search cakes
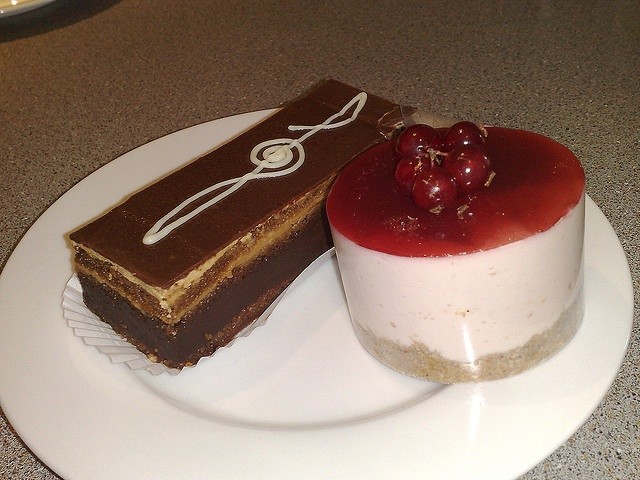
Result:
[69,78,418,371]
[325,120,587,386]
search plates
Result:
[1,109,635,479]
